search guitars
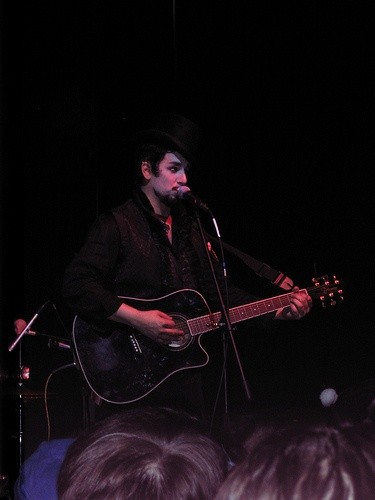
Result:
[70,274,347,406]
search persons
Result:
[56,398,375,500]
[62,132,314,414]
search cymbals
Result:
[0,386,64,400]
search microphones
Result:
[177,186,209,211]
[9,305,43,351]
